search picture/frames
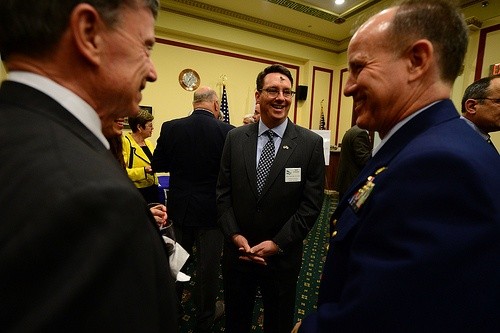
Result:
[115,106,152,129]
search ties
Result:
[256,130,276,196]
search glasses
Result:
[473,97,500,103]
[258,88,297,98]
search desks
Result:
[325,147,342,189]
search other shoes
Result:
[180,287,192,306]
[196,300,224,326]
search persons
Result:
[333,124,373,201]
[156,84,237,333]
[118,106,161,205]
[100,114,167,231]
[215,63,326,333]
[0,0,186,332]
[290,0,500,332]
[253,104,261,123]
[243,114,254,124]
[460,75,499,131]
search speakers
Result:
[297,86,308,100]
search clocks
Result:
[179,68,201,91]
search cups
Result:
[147,202,164,233]
[159,219,176,261]
